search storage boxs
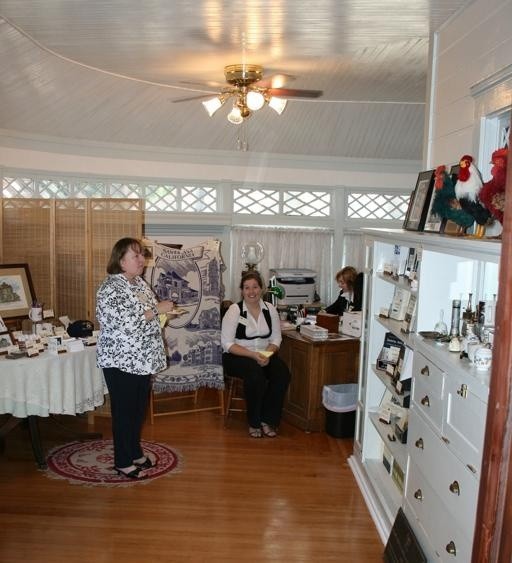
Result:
[342,309,361,337]
[314,312,339,333]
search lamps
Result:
[204,91,288,124]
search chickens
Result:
[477,147,509,240]
[428,165,475,239]
[452,153,491,239]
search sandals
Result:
[261,422,277,437]
[249,427,262,438]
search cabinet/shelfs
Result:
[347,226,504,562]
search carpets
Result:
[43,435,186,488]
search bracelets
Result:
[151,304,160,317]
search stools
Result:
[223,371,248,426]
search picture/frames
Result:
[0,263,37,322]
[402,168,437,231]
[420,172,457,234]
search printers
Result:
[270,268,318,306]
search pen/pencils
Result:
[297,311,304,318]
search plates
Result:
[167,309,188,314]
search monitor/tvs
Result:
[305,306,321,315]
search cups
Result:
[419,331,439,338]
[295,316,305,326]
[278,311,288,321]
[28,307,69,337]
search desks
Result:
[0,320,109,471]
[272,320,360,435]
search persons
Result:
[323,266,362,316]
[221,271,290,438]
[96,237,181,481]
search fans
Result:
[168,62,326,124]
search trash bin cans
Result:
[323,383,364,438]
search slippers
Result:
[114,466,148,479]
[133,456,157,468]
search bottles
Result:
[435,292,497,371]
